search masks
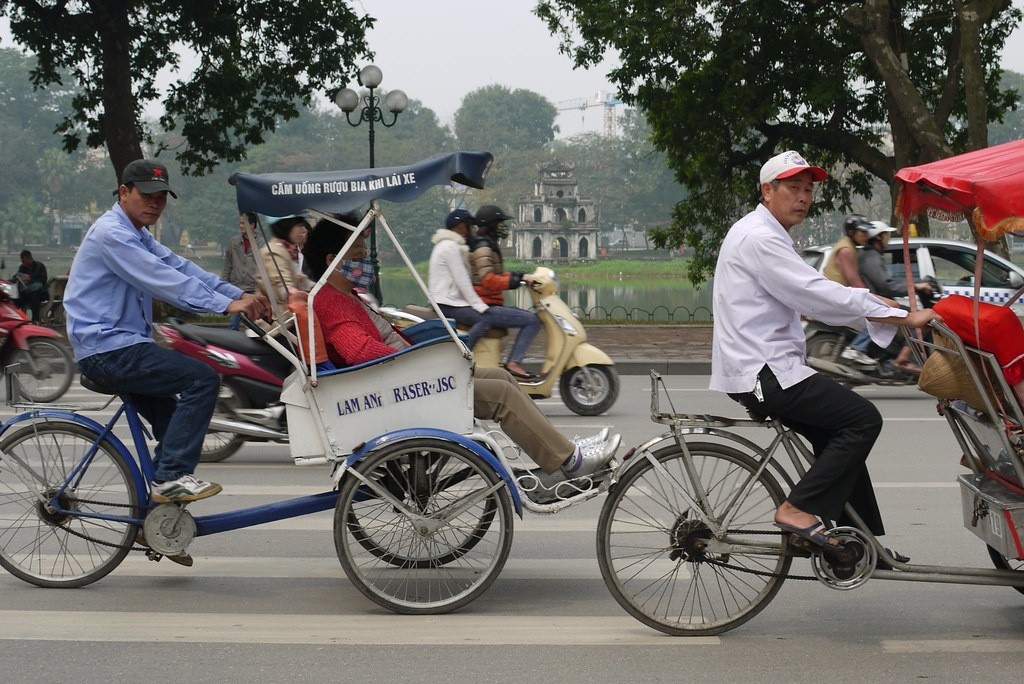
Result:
[331,255,374,286]
[496,222,510,239]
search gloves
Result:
[523,274,543,288]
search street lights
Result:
[334,64,408,305]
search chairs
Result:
[897,257,938,280]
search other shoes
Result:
[34,320,41,326]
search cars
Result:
[797,238,1024,323]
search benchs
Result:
[36,273,70,328]
[932,295,1024,442]
[287,291,478,456]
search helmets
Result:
[846,217,876,232]
[475,205,513,226]
[867,220,899,239]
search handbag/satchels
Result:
[15,276,51,306]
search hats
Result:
[760,149,828,186]
[123,158,177,199]
[446,209,477,230]
[915,318,999,413]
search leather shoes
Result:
[501,363,542,381]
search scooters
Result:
[0,260,75,403]
[151,283,380,463]
[381,266,620,417]
[802,274,945,390]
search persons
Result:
[822,214,880,364]
[9,250,48,324]
[220,212,272,330]
[428,210,491,352]
[471,203,540,380]
[257,218,316,320]
[709,151,942,569]
[300,214,622,484]
[62,161,271,504]
[858,220,933,371]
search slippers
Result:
[867,547,912,562]
[773,520,846,550]
[890,360,922,376]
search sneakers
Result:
[841,345,878,366]
[557,427,623,484]
[150,475,223,503]
[135,527,194,567]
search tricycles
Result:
[0,149,621,617]
[593,136,1024,639]
